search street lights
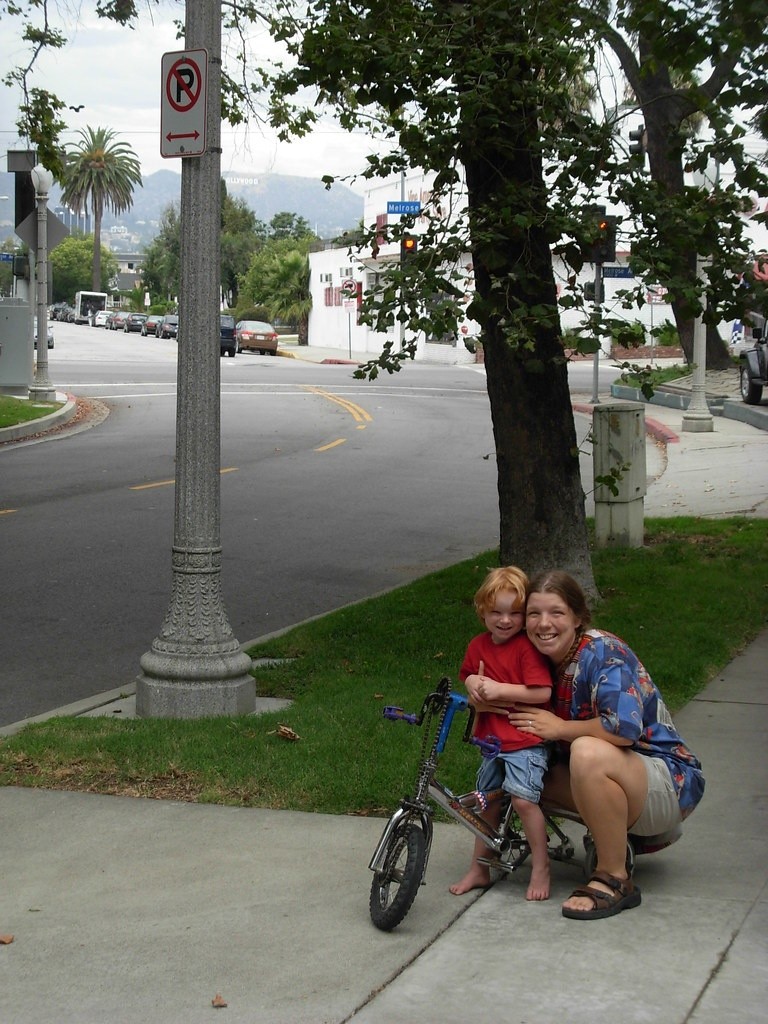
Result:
[28,163,57,391]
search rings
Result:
[528,720,532,726]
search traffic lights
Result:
[628,123,646,168]
[581,214,617,263]
[400,233,419,276]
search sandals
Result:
[559,867,643,920]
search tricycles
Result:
[366,676,636,932]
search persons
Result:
[448,566,706,919]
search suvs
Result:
[220,315,241,358]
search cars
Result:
[235,320,279,356]
[738,316,768,405]
[48,290,179,341]
